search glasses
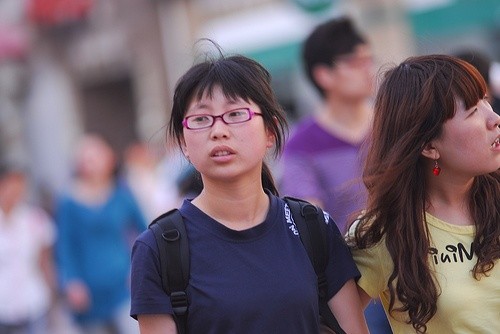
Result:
[181,106,264,130]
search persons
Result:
[344,49,500,334]
[128,38,371,334]
[283,13,393,334]
[0,128,153,334]
